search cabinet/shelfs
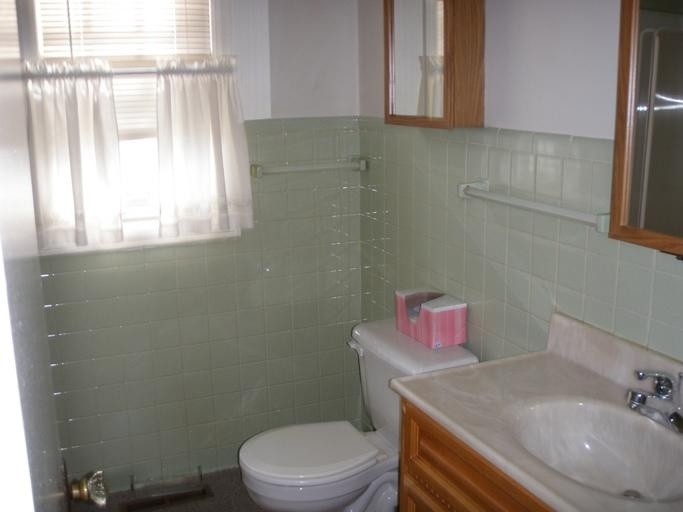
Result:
[396,397,557,511]
[609,1,683,255]
[385,0,484,128]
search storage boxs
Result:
[391,286,469,352]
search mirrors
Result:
[390,4,446,117]
[626,0,682,244]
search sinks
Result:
[508,396,683,503]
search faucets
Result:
[626,369,683,432]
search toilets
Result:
[239,318,479,512]
